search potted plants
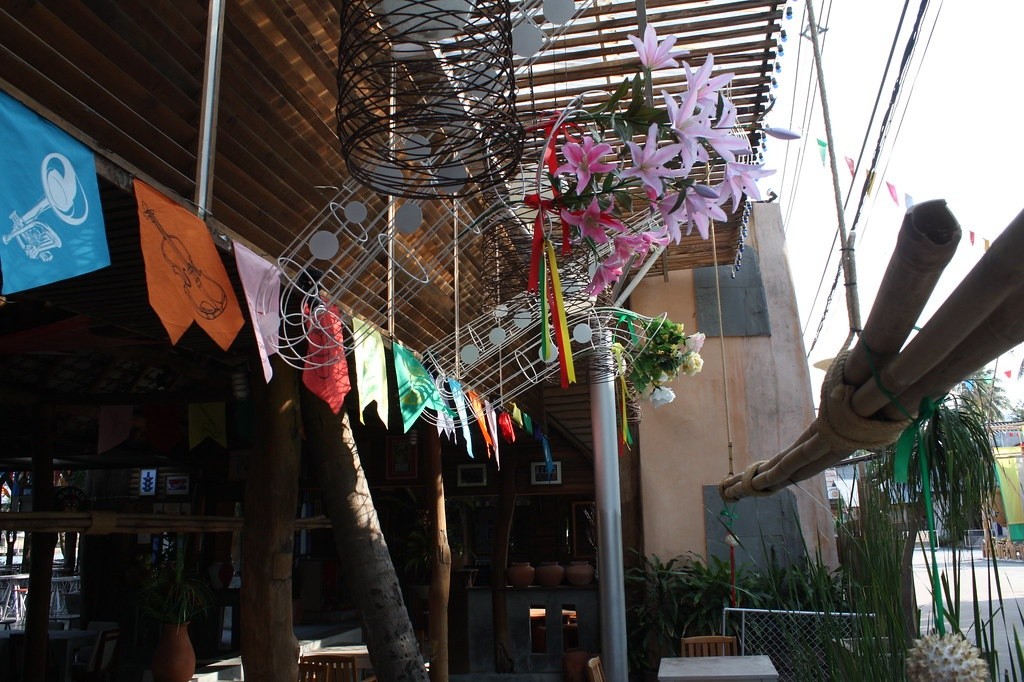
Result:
[144,566,214,682]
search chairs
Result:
[298,655,377,682]
[414,588,426,652]
[588,657,606,682]
[680,637,739,656]
[73,627,124,682]
[74,622,122,682]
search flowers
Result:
[612,313,705,407]
[527,31,782,294]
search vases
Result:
[507,562,536,587]
[536,562,566,586]
[532,623,547,653]
[562,648,588,682]
[450,571,473,597]
[567,562,594,586]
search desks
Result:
[659,655,780,682]
[8,631,98,682]
[310,645,373,681]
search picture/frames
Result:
[164,474,189,496]
[457,465,491,487]
[385,437,418,480]
[529,460,567,487]
